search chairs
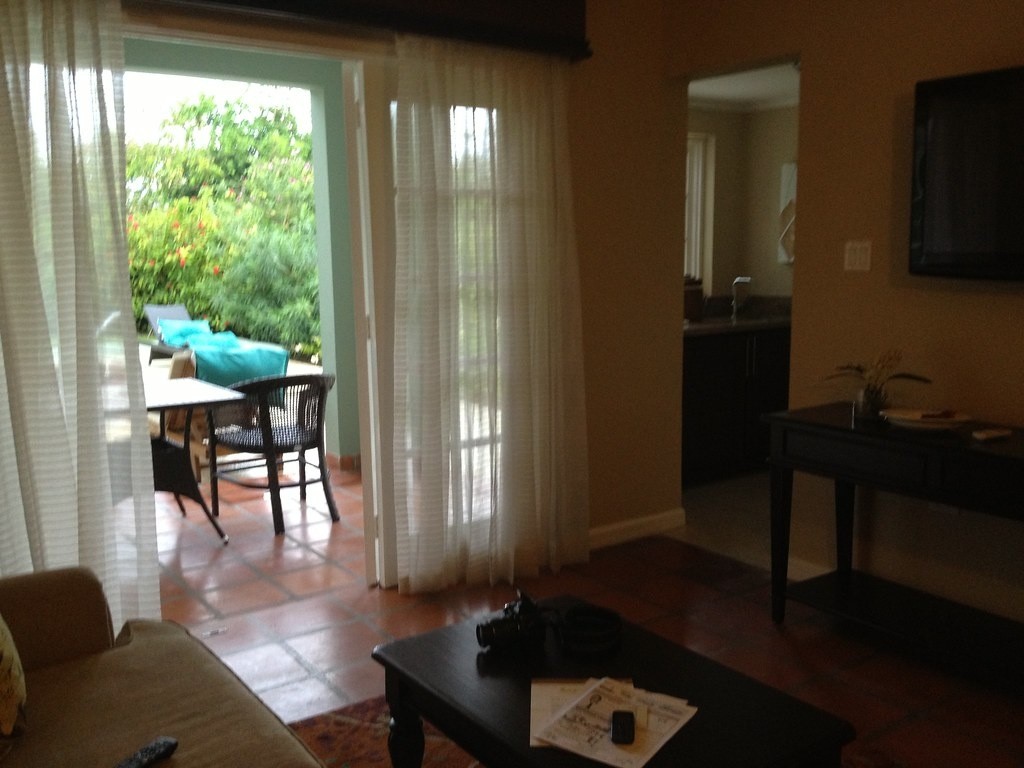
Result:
[205,372,342,537]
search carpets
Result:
[290,692,482,768]
[200,474,295,505]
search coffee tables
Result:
[372,593,859,768]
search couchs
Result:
[0,563,329,768]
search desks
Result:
[770,400,1024,696]
[101,375,246,544]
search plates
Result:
[880,409,977,428]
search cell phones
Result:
[611,711,634,744]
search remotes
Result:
[111,736,179,768]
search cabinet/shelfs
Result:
[682,328,791,485]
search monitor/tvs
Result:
[908,65,1024,282]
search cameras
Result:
[477,586,561,647]
